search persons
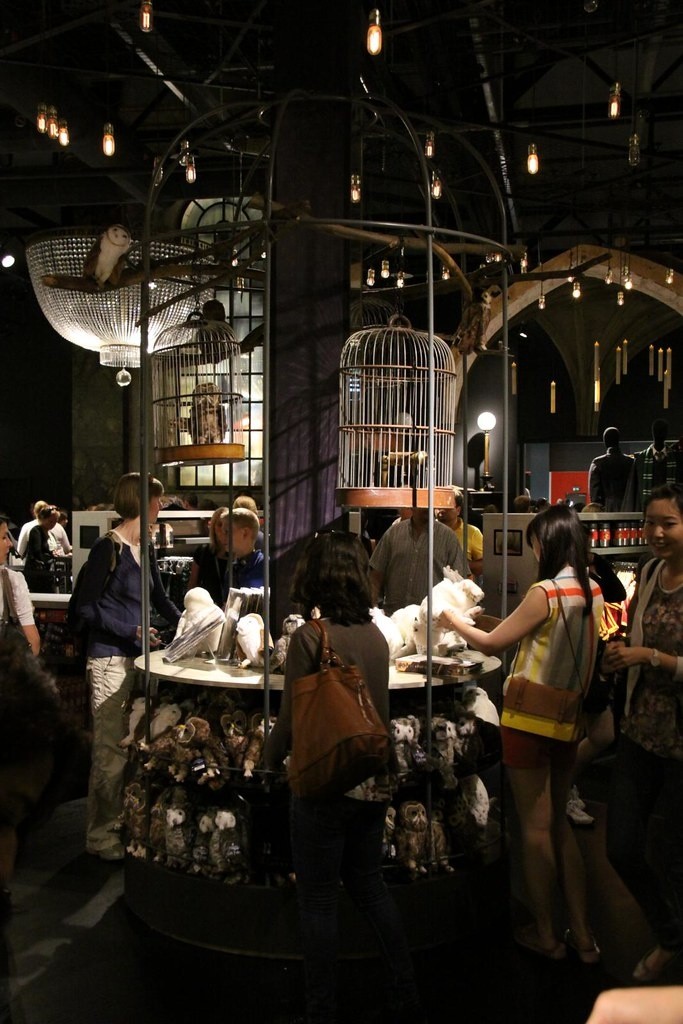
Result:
[436,504,600,962]
[588,427,634,512]
[582,522,631,748]
[0,619,81,897]
[0,513,42,657]
[434,488,483,575]
[368,479,472,618]
[16,501,73,593]
[180,490,197,509]
[477,505,498,535]
[361,510,373,558]
[262,530,422,1024]
[392,485,413,524]
[185,497,264,612]
[69,473,183,862]
[512,497,606,512]
[197,498,219,510]
[620,419,683,512]
[604,485,683,979]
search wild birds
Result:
[172,586,275,670]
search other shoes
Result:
[632,945,681,981]
[87,840,124,860]
[275,1012,334,1024]
[364,1003,429,1024]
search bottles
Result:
[583,519,654,548]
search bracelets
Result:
[449,617,456,628]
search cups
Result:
[157,555,194,574]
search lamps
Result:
[477,411,496,491]
[2,1,674,413]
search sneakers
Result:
[567,795,595,824]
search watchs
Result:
[650,646,661,668]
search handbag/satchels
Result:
[500,675,585,744]
[287,619,396,798]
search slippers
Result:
[564,928,601,964]
[515,923,568,960]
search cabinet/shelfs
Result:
[118,642,530,962]
